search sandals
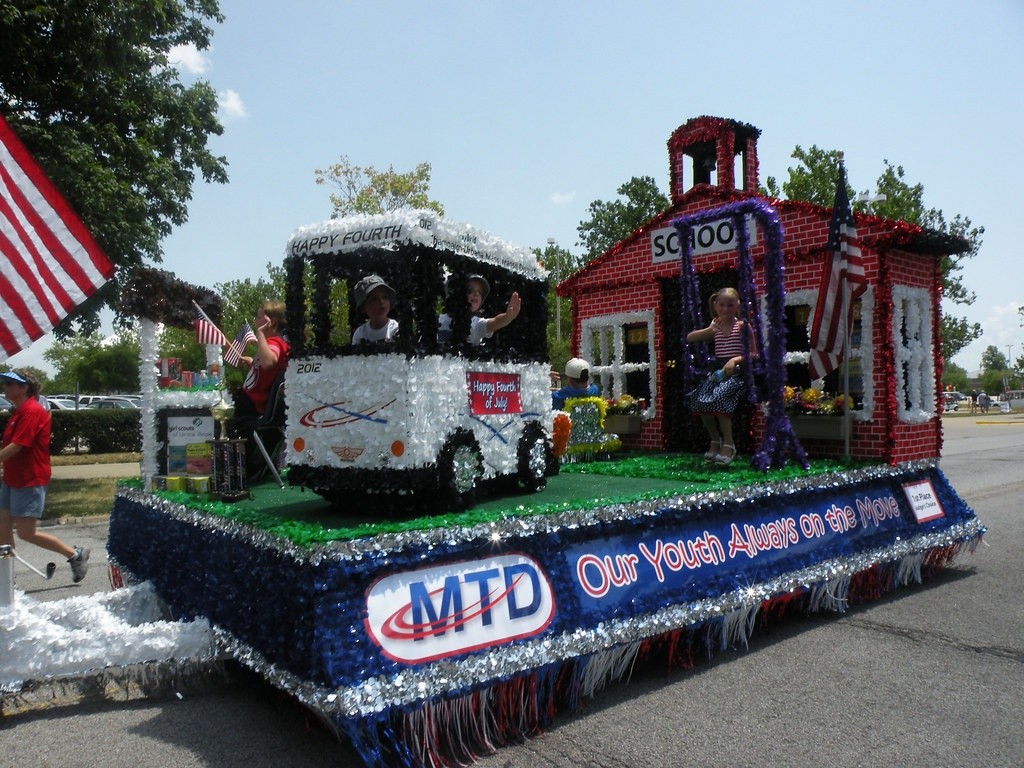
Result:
[704,437,722,462]
[715,441,738,465]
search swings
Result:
[679,212,765,406]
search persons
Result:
[979,390,986,413]
[436,274,521,345]
[983,393,990,413]
[0,368,89,583]
[687,288,758,465]
[231,300,288,435]
[551,358,598,411]
[352,275,400,344]
[968,389,982,414]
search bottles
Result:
[199,370,219,385]
[713,364,740,381]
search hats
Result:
[462,272,491,308]
[0,372,27,383]
[353,274,400,319]
[980,390,985,393]
[564,357,591,379]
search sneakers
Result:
[66,544,91,582]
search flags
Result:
[807,162,868,381]
[196,309,227,345]
[0,111,115,366]
[223,319,258,367]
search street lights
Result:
[1005,344,1014,368]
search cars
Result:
[943,391,967,412]
[0,393,145,411]
[975,397,1001,407]
